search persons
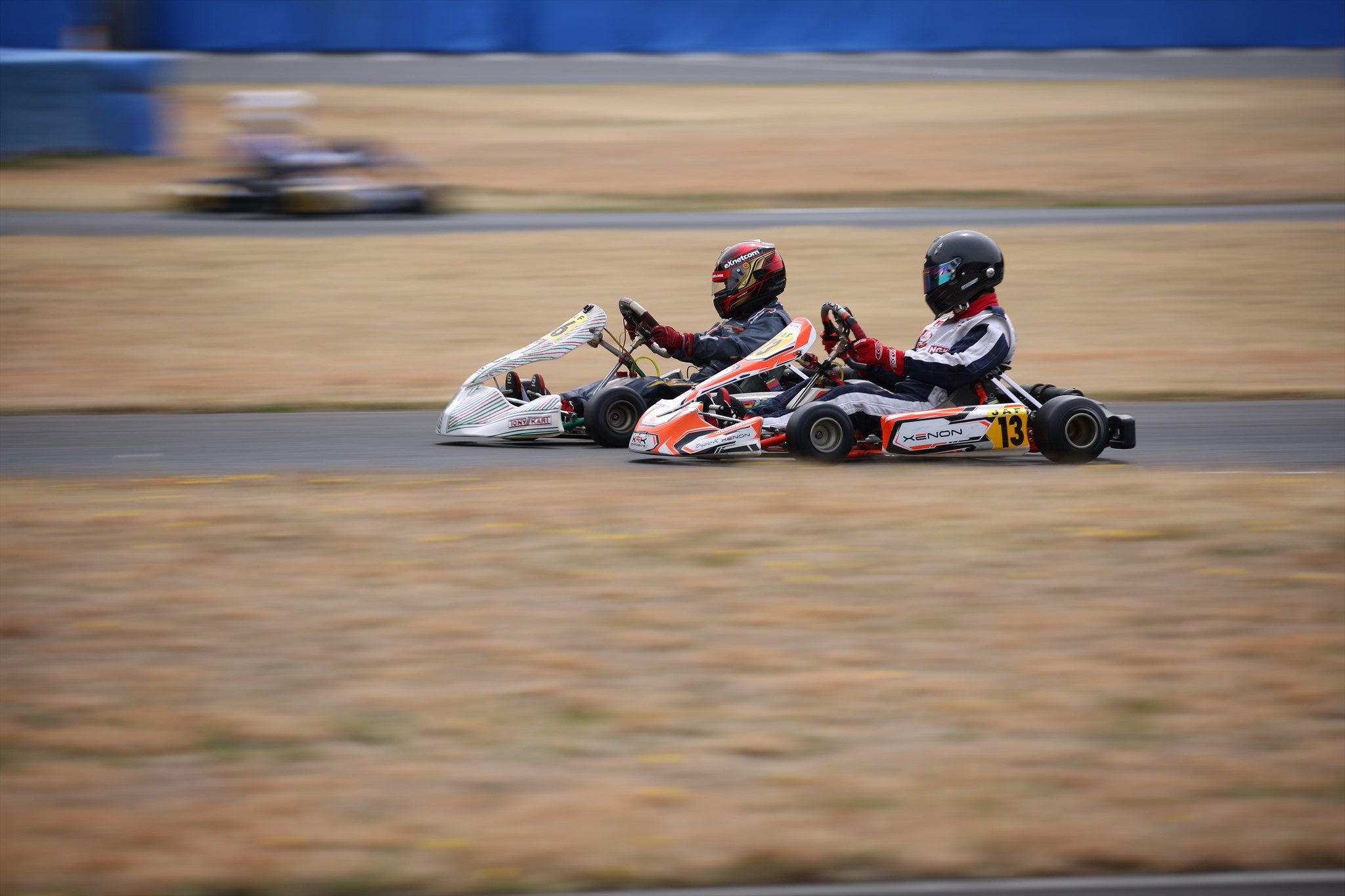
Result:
[696,230,1017,443]
[506,240,792,418]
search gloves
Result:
[625,320,655,349]
[850,337,905,377]
[819,330,852,360]
[651,325,693,356]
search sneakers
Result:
[697,393,718,427]
[717,387,752,429]
[531,374,574,416]
[505,371,530,407]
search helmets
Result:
[711,239,787,319]
[923,230,1005,321]
